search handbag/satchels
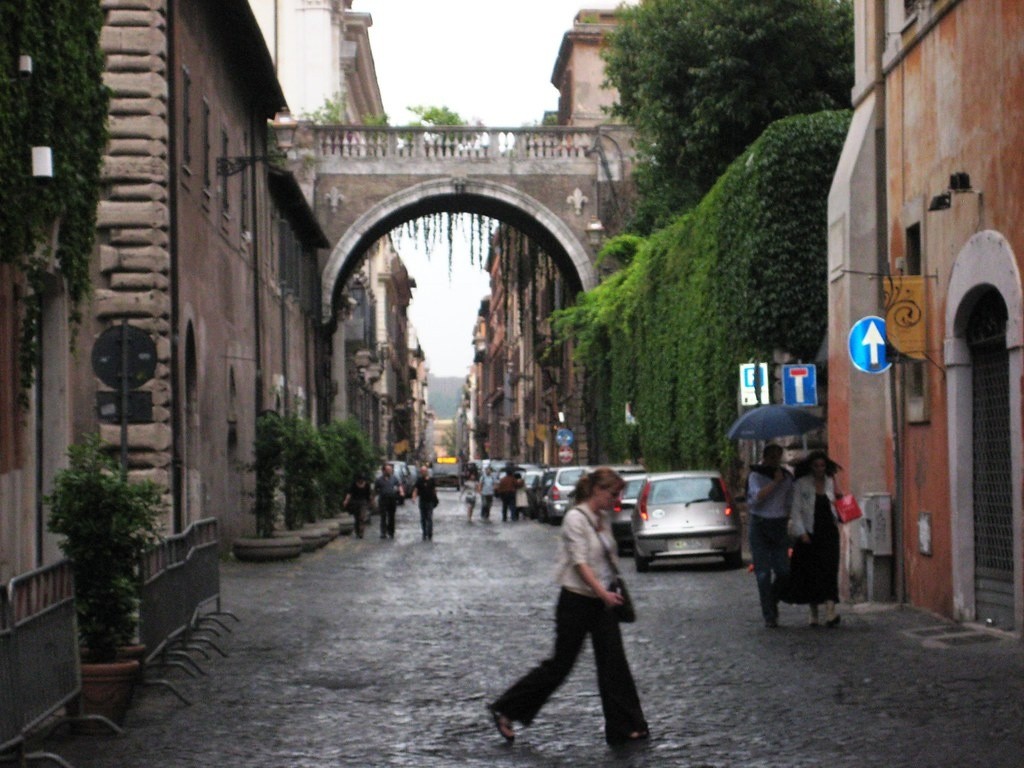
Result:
[609,577,637,623]
[834,493,862,523]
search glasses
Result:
[608,489,620,498]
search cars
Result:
[374,461,418,503]
[610,473,668,557]
[626,468,742,572]
[495,464,599,524]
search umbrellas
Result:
[729,404,824,468]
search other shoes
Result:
[491,708,516,743]
[824,611,841,626]
[807,618,819,625]
[631,730,647,740]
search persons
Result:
[460,463,496,523]
[778,452,847,626]
[426,461,433,477]
[489,469,652,745]
[375,464,405,538]
[342,473,373,538]
[497,470,530,522]
[747,444,795,623]
[413,466,437,540]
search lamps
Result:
[585,210,604,252]
[216,106,300,177]
[927,172,981,212]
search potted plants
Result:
[41,428,170,730]
